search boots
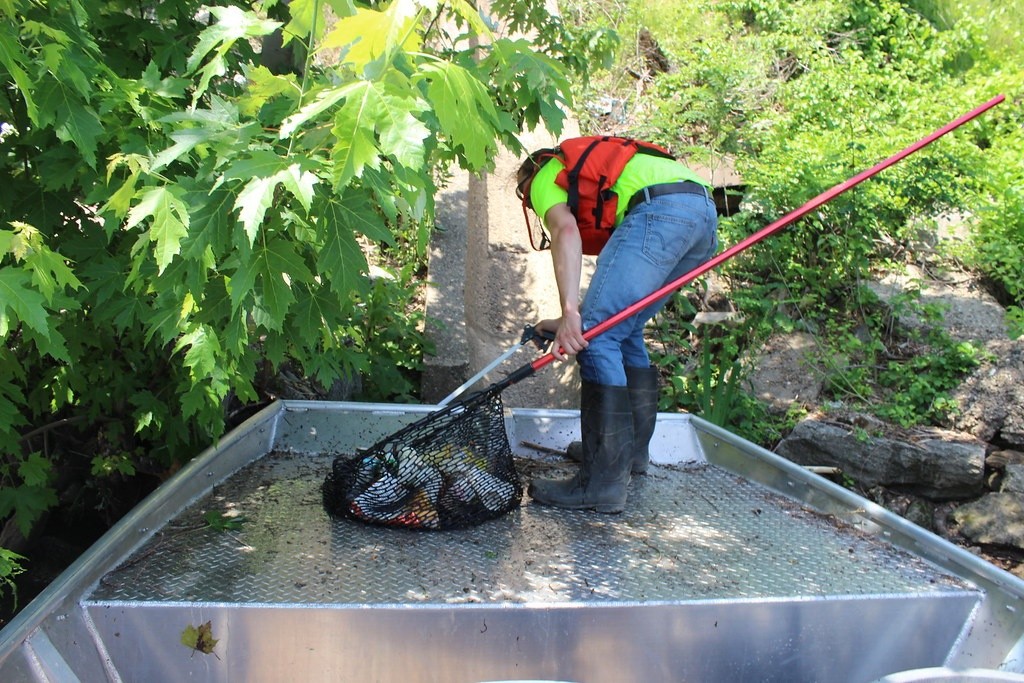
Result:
[566,363,658,473]
[527,380,636,514]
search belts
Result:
[628,182,715,212]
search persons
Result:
[515,135,719,515]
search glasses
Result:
[515,168,533,201]
